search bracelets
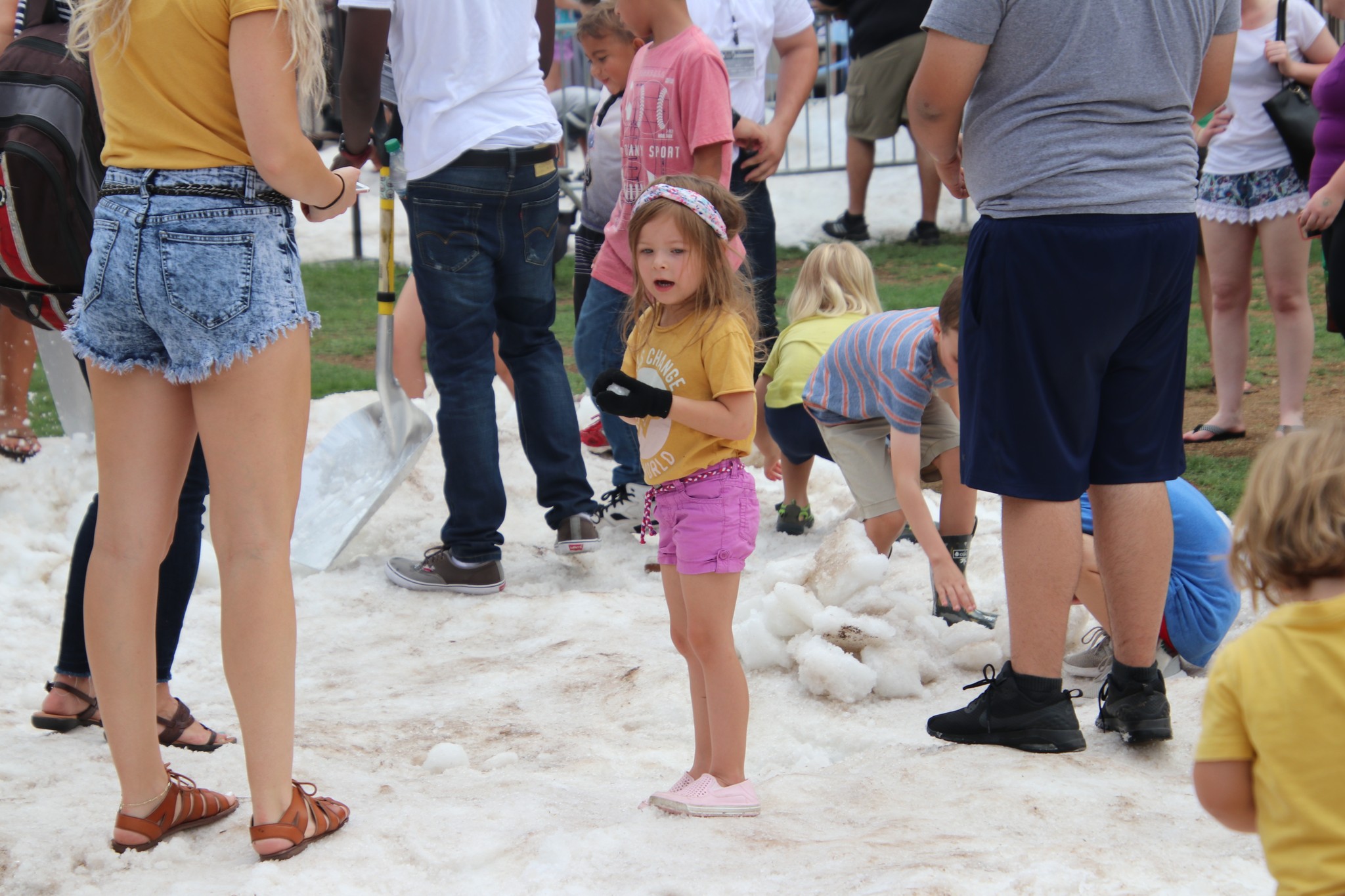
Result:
[338,133,374,163]
[314,173,345,209]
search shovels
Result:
[289,101,437,576]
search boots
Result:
[933,534,999,629]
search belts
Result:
[450,144,558,168]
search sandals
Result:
[157,696,237,752]
[111,762,238,854]
[31,680,102,732]
[249,778,350,861]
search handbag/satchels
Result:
[1261,0,1320,182]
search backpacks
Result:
[0,0,105,334]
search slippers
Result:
[1182,424,1248,443]
[1277,424,1304,438]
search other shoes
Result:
[774,500,814,535]
[0,429,41,461]
[895,523,917,543]
[637,771,695,811]
[579,414,612,454]
[652,772,761,817]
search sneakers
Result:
[1062,625,1180,680]
[554,516,602,556]
[384,546,506,596]
[600,482,661,535]
[1095,669,1173,745]
[908,219,940,246]
[821,212,870,241]
[926,660,1086,754]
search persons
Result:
[908,0,1241,753]
[580,0,654,455]
[0,0,68,454]
[1298,0,1345,334]
[803,274,996,629]
[575,0,747,523]
[686,0,819,381]
[329,0,602,595]
[1182,0,1340,442]
[32,432,238,752]
[1192,427,1345,896]
[67,0,361,861]
[755,242,884,536]
[590,175,761,818]
[816,0,941,243]
[1063,477,1241,674]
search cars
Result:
[811,17,851,89]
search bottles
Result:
[384,138,406,196]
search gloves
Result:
[591,367,673,419]
